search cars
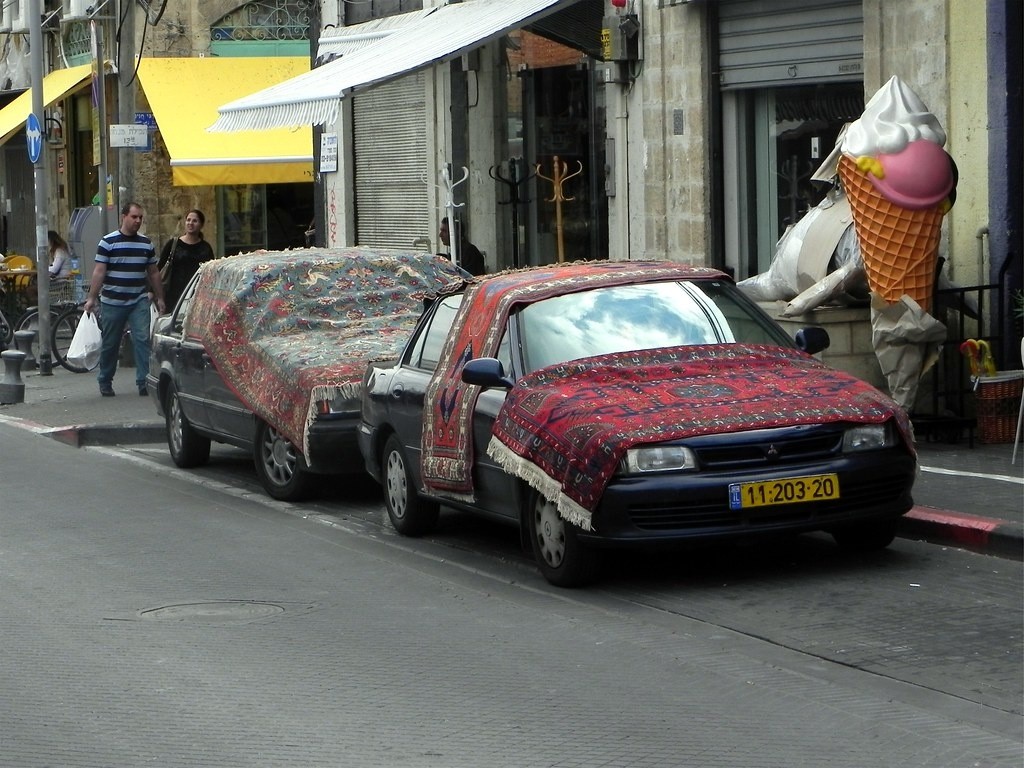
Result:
[146,247,473,500]
[356,260,917,587]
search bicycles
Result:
[0,276,133,373]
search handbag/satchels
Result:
[66,309,102,370]
[156,236,179,287]
[149,301,160,339]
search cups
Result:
[21,265,26,270]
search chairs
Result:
[2,255,34,288]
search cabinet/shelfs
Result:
[223,210,264,247]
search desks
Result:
[0,269,37,323]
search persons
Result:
[439,217,484,277]
[84,202,166,396]
[156,210,214,315]
[48,230,71,283]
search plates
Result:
[11,268,28,272]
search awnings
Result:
[134,56,317,187]
[205,0,606,134]
[0,59,110,146]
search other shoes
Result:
[138,384,148,396]
[100,382,115,396]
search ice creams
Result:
[836,74,958,315]
